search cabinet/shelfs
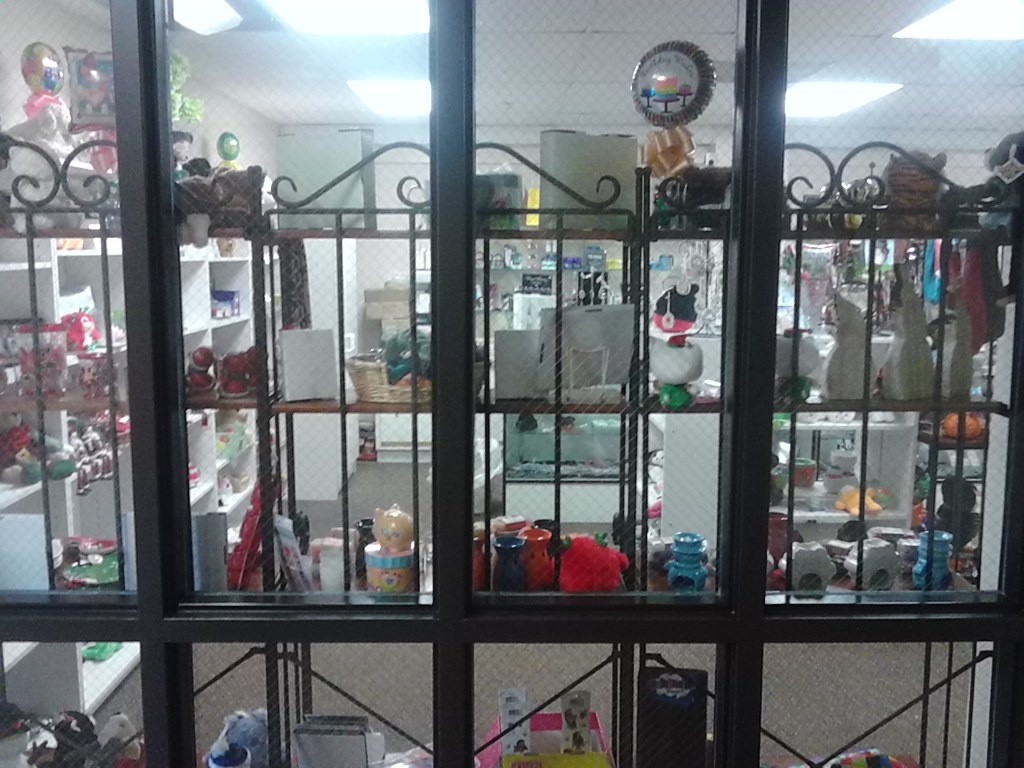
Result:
[637,336,920,555]
[0,143,1024,768]
[0,158,281,768]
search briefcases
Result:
[637,652,707,767]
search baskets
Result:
[346,354,435,403]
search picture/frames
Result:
[64,46,115,135]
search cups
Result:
[795,458,817,486]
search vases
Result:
[492,536,525,592]
[664,531,708,604]
[474,537,484,590]
[912,530,953,592]
[519,528,553,590]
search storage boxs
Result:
[364,289,411,319]
[381,320,410,331]
[506,413,629,483]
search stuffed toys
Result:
[965,129,1024,239]
[204,709,269,768]
[61,303,102,354]
[84,711,141,767]
[878,148,947,229]
[0,413,71,485]
[834,485,882,520]
[546,532,630,593]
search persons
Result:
[64,423,116,497]
